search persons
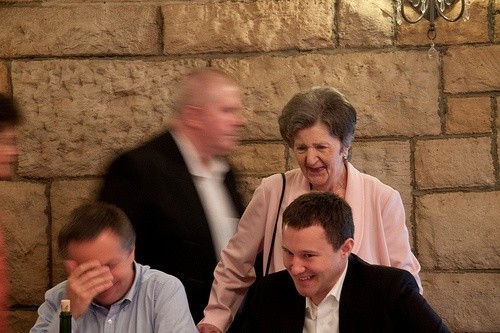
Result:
[96,68,273,332]
[195,86,424,333]
[0,93,23,331]
[28,202,198,333]
[226,192,451,333]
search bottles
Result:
[59,299,72,333]
